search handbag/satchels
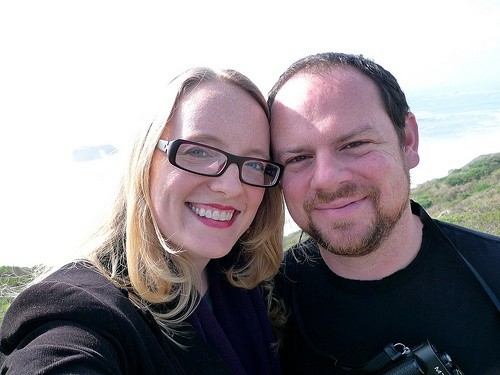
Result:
[358,338,465,375]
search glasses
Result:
[155,138,286,189]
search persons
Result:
[0,66,280,375]
[267,53,500,375]
